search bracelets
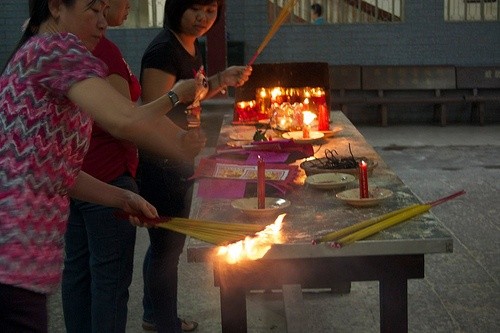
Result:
[217,71,227,94]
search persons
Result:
[1,0,213,332]
[309,3,327,25]
[58,0,207,332]
[136,0,253,333]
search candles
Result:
[359,160,370,199]
[302,111,317,138]
[231,85,331,131]
[257,155,266,210]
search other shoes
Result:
[142,317,195,333]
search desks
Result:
[186,111,455,333]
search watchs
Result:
[167,90,179,109]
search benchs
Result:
[235,62,500,128]
